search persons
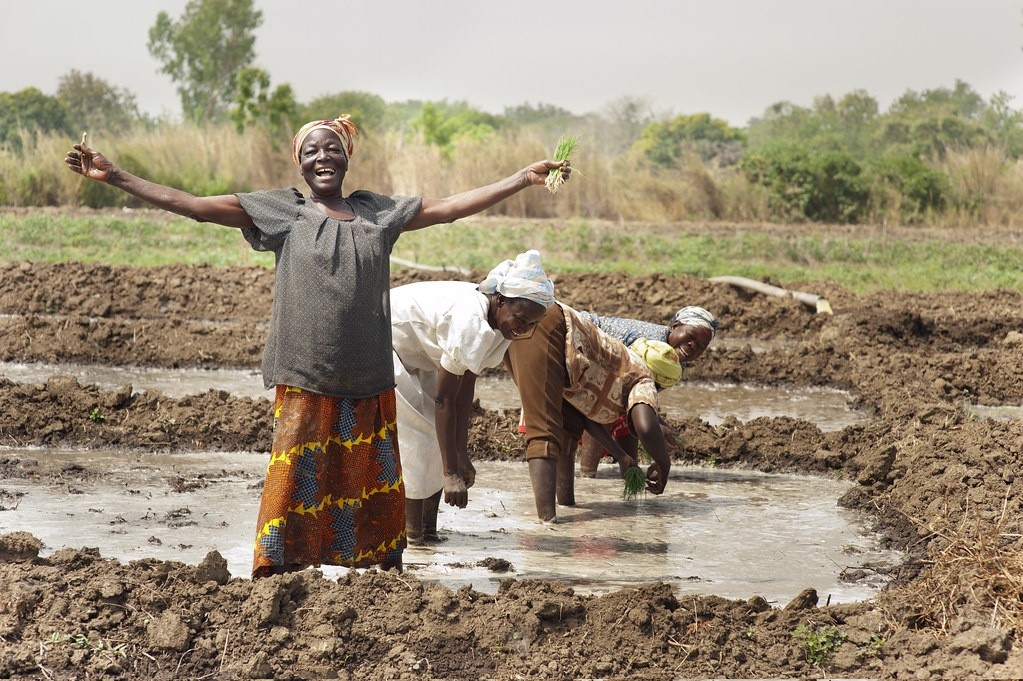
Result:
[580,307,718,478]
[64,114,571,582]
[502,301,682,525]
